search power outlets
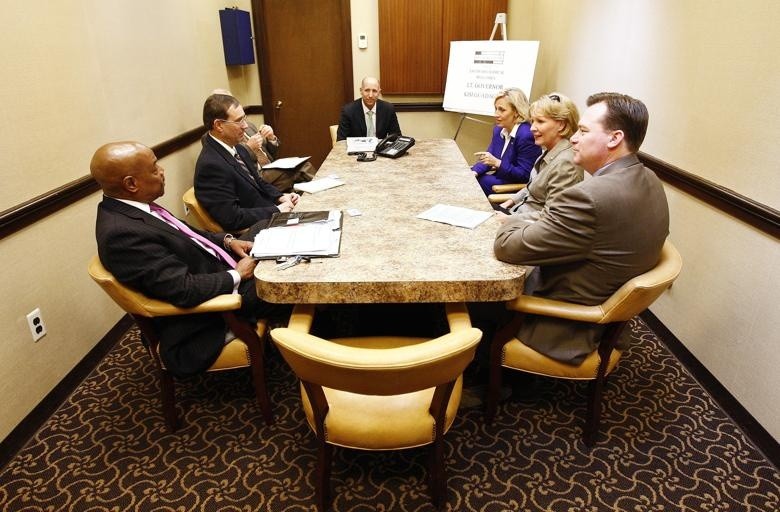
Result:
[24,305,49,346]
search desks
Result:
[253,136,527,422]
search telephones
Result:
[348,134,415,161]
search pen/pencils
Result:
[252,254,254,258]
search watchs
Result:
[227,237,237,246]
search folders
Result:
[251,210,344,259]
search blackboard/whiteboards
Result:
[442,39,540,117]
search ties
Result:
[367,111,375,137]
[148,204,239,269]
[236,154,260,184]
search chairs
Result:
[267,299,485,511]
[181,184,252,238]
[88,251,274,435]
[328,121,340,143]
[487,240,684,448]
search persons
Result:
[201,88,318,198]
[494,93,669,366]
[90,142,290,378]
[471,89,544,195]
[336,77,404,141]
[194,94,300,241]
[486,95,585,215]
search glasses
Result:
[218,116,248,124]
[547,95,559,103]
